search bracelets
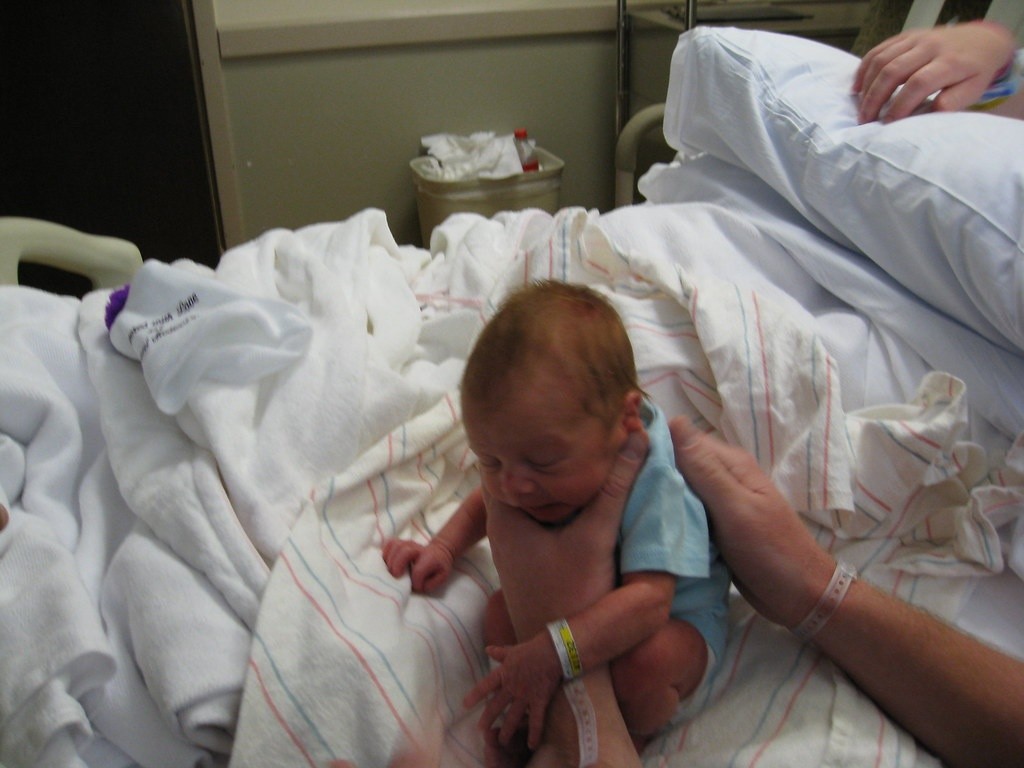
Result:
[969,20,1020,111]
[547,617,583,680]
[791,547,855,646]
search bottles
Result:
[513,127,538,172]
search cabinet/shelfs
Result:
[617,0,886,141]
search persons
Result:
[849,19,1024,126]
[380,278,1023,767]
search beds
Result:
[0,104,1023,768]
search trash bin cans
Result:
[408,128,566,251]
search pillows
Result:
[640,28,1023,450]
